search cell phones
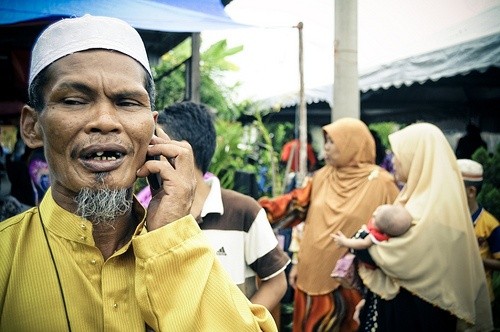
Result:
[146,152,161,190]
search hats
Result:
[28,14,153,97]
[457,158,483,182]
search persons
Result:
[456,159,500,307]
[352,123,494,332]
[257,117,401,332]
[331,204,412,249]
[278,127,315,173]
[455,122,488,160]
[0,13,278,332]
[154,102,291,312]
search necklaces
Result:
[37,204,73,332]
